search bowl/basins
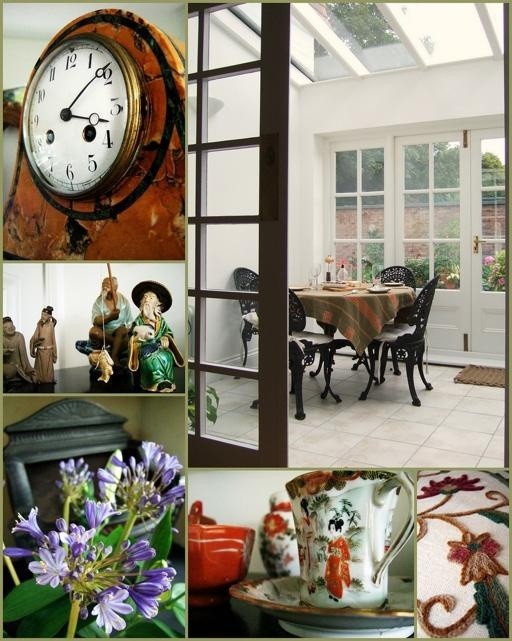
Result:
[187,501,255,608]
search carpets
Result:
[453,365,507,387]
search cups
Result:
[371,264,382,288]
[286,467,416,609]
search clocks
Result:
[1,7,188,261]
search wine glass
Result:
[312,264,321,292]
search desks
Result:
[188,572,415,637]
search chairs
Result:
[349,263,419,376]
[249,279,339,423]
[359,275,442,407]
[232,264,259,379]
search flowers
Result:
[1,442,186,639]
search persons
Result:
[29,306,57,383]
[1,316,38,385]
[324,519,352,600]
[127,279,181,393]
[87,276,134,369]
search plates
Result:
[231,578,415,640]
[368,287,391,293]
[289,287,305,291]
[383,283,404,287]
